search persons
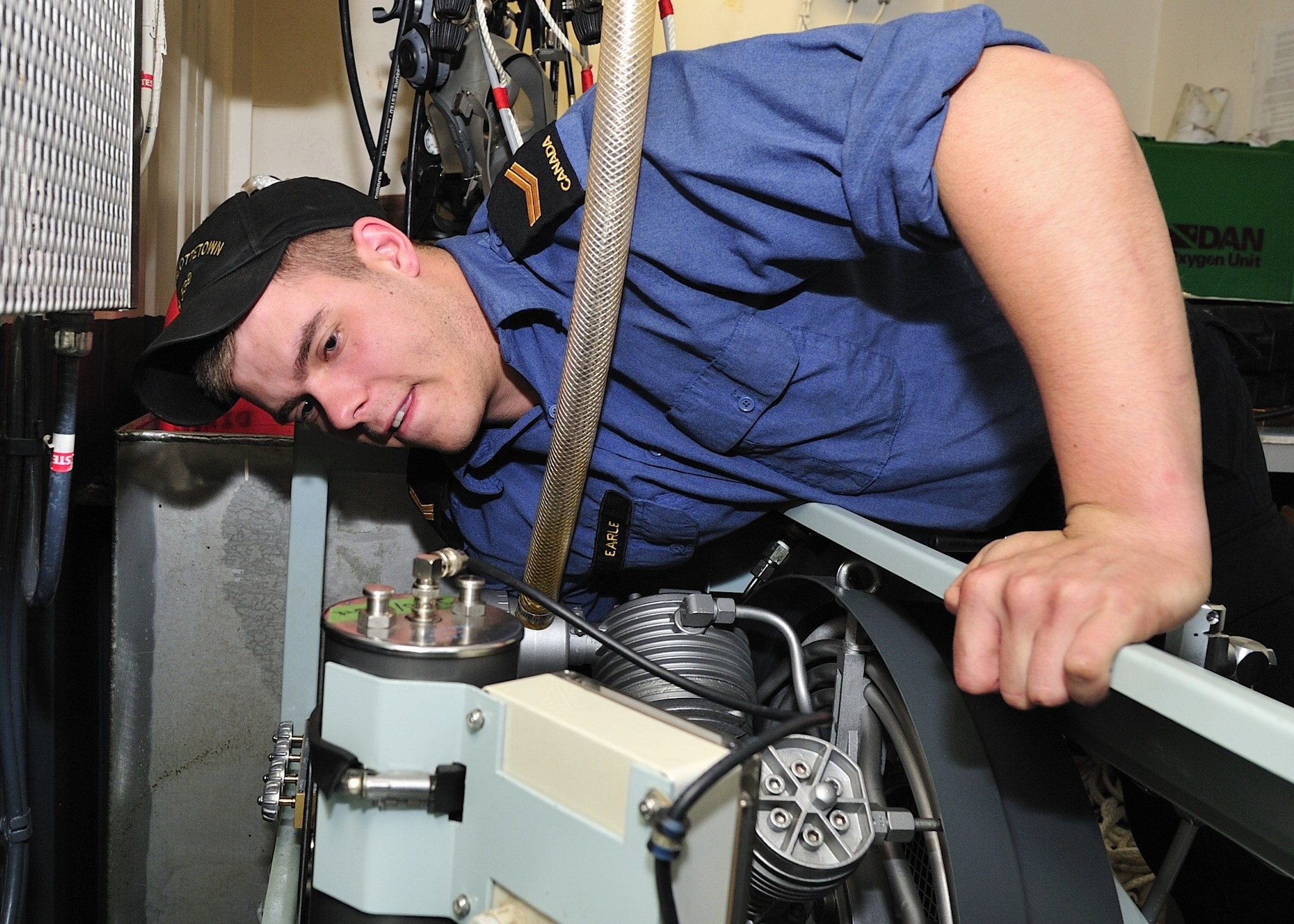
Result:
[134,4,1294,924]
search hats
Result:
[129,173,395,428]
